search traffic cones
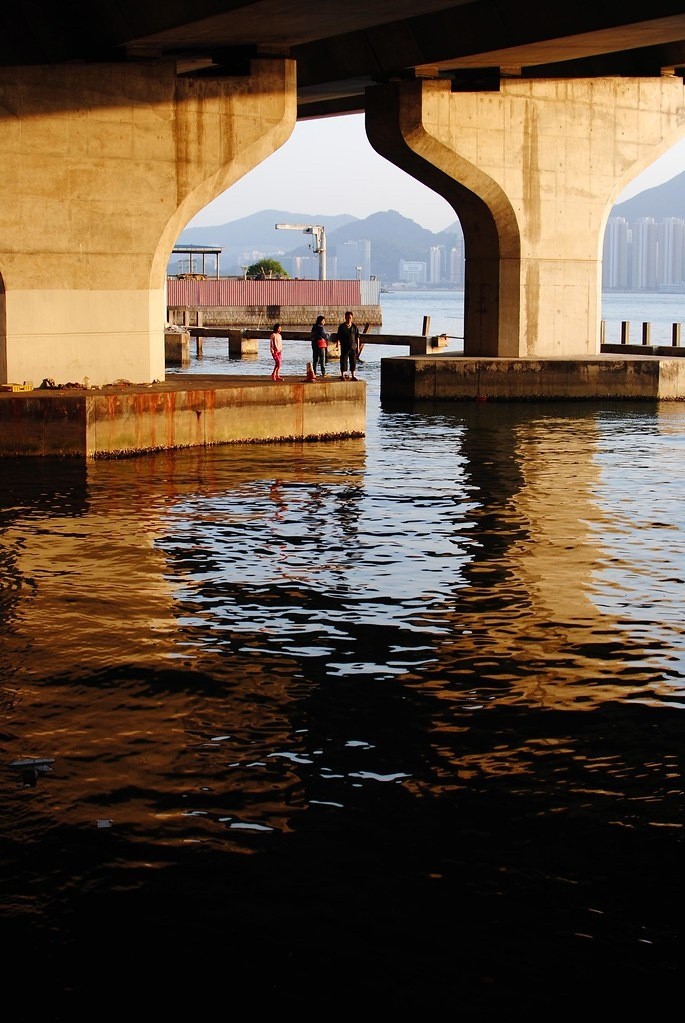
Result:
[305,362,317,382]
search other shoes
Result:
[351,377,359,381]
[272,374,276,381]
[341,377,346,381]
[276,376,284,381]
[322,374,330,378]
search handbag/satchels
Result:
[318,339,327,348]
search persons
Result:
[310,316,331,377]
[270,323,284,381]
[336,312,360,381]
